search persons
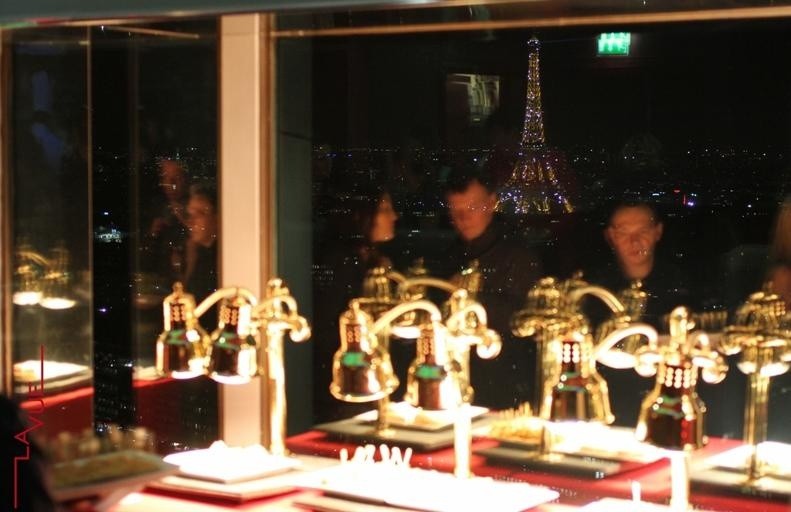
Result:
[180,180,221,323]
[588,199,701,429]
[310,177,421,420]
[714,203,791,439]
[147,138,202,276]
[438,158,548,407]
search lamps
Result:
[157,269,790,510]
